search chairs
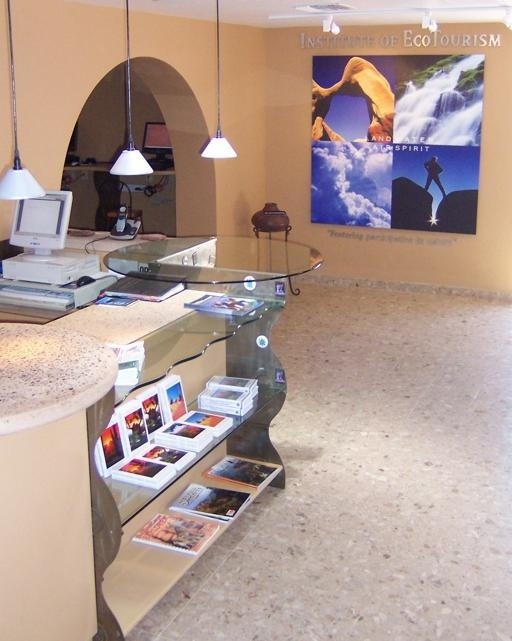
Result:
[97,179,144,232]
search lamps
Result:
[322,15,341,35]
[200,0,238,158]
[0,0,45,200]
[420,10,438,31]
[109,0,154,175]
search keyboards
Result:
[1,286,75,304]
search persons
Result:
[424,156,447,198]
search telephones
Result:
[110,200,142,240]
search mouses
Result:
[76,275,93,289]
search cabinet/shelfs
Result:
[45,289,285,639]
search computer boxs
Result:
[2,252,101,284]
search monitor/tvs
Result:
[142,122,173,167]
[9,190,75,261]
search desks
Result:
[0,319,118,640]
[64,158,176,176]
[105,233,323,285]
[0,306,90,323]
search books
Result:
[130,454,283,556]
[183,294,265,317]
[95,373,260,489]
